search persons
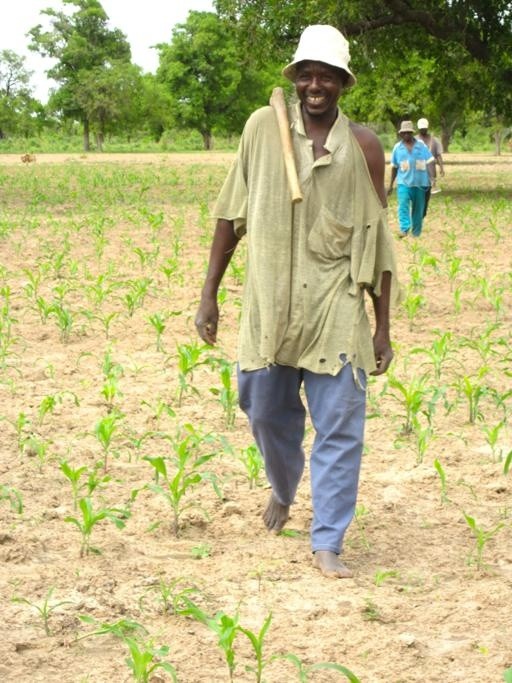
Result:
[386,121,437,238]
[414,117,445,193]
[193,24,401,580]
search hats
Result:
[282,25,357,88]
[398,121,416,134]
[418,118,428,130]
